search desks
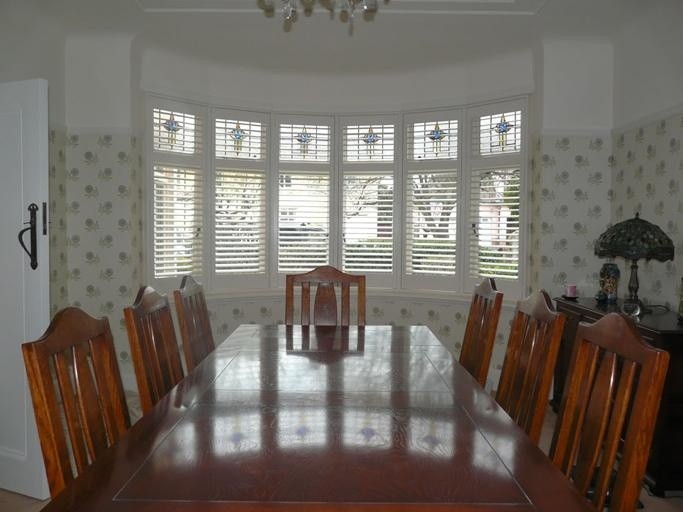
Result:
[59,322,616,509]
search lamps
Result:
[594,209,674,315]
[257,0,390,23]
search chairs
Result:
[171,273,219,375]
[550,311,669,510]
[21,306,135,505]
[279,264,369,326]
[492,288,567,453]
[456,277,506,393]
[120,284,188,416]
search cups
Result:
[566,285,577,296]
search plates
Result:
[562,296,579,300]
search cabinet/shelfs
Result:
[548,295,681,496]
